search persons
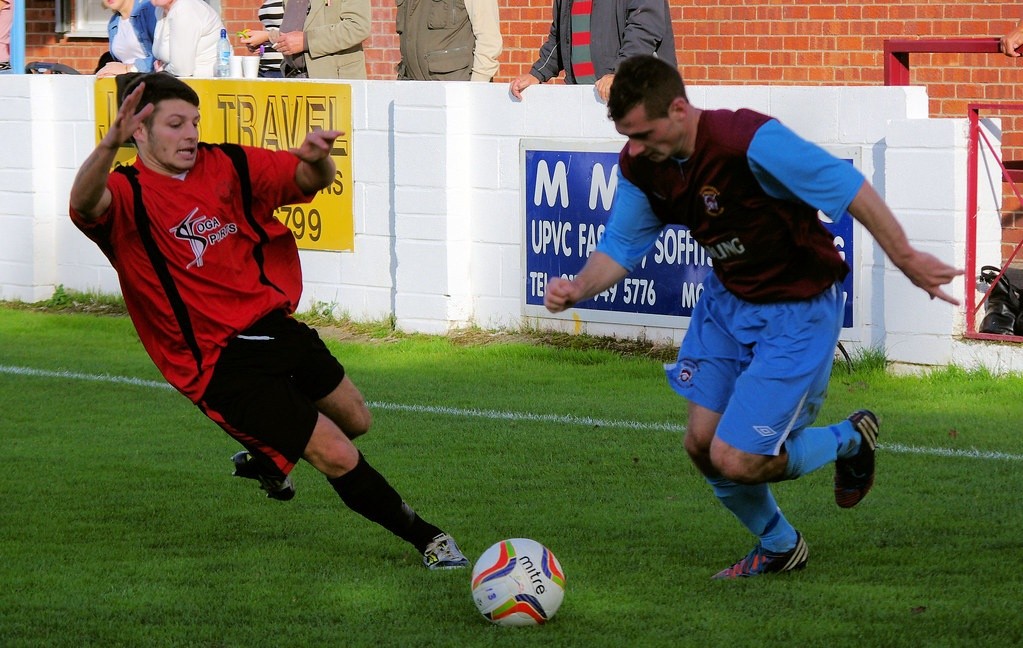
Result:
[544,55,965,580]
[96,0,156,77]
[240,0,286,78]
[0,0,14,70]
[396,0,503,82]
[274,0,371,79]
[69,73,471,570]
[151,0,234,78]
[510,0,677,103]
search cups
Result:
[231,56,243,77]
[243,56,261,77]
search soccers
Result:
[471,538,566,626]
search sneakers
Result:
[710,529,808,582]
[834,409,879,508]
[230,450,295,501]
[422,532,472,570]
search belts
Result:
[285,67,307,75]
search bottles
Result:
[216,29,231,78]
